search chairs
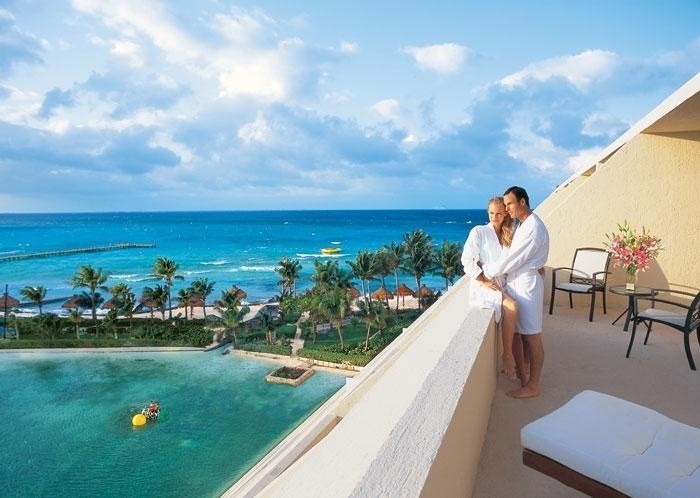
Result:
[521,388,699,497]
[625,289,700,369]
[549,247,612,320]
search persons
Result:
[476,185,550,398]
[143,400,160,421]
[461,196,528,388]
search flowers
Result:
[601,218,665,273]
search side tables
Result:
[608,284,658,330]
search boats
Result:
[321,248,342,254]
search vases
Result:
[626,268,638,290]
